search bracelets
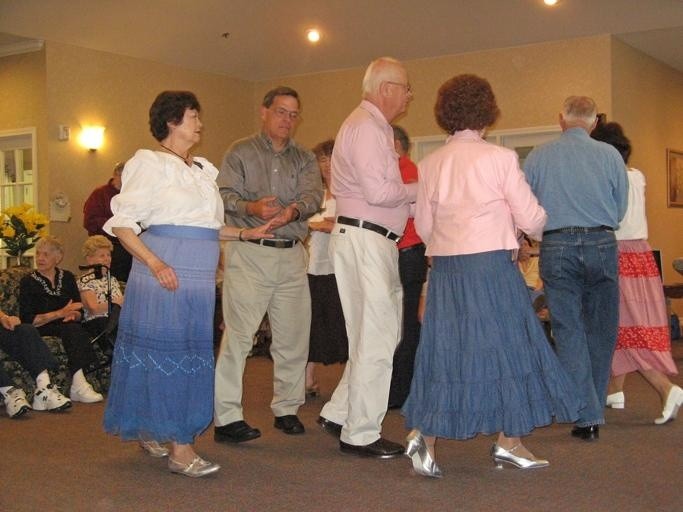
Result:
[296,209,300,222]
[237,228,247,242]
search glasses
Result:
[386,81,410,91]
[275,107,298,120]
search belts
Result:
[543,227,611,235]
[247,239,297,247]
[336,215,401,244]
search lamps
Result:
[82,124,105,153]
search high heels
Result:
[490,443,549,470]
[403,429,442,479]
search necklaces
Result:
[161,145,192,168]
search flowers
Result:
[0,204,49,259]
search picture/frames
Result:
[665,146,682,208]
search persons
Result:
[387,125,427,414]
[213,87,324,445]
[302,139,348,398]
[74,236,125,323]
[0,309,72,419]
[522,92,629,445]
[315,54,417,461]
[583,111,682,428]
[18,236,117,404]
[518,236,548,322]
[100,87,280,478]
[82,159,131,283]
[403,74,588,480]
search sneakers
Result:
[32,384,72,413]
[70,384,103,404]
[4,385,31,419]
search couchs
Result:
[0,265,125,409]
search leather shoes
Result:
[606,392,624,409]
[137,434,169,458]
[273,415,304,435]
[316,417,405,459]
[571,424,598,442]
[214,421,260,442]
[167,454,221,478]
[654,385,682,425]
